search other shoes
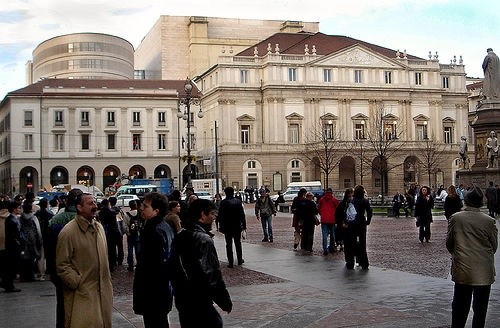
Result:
[262,239,268,242]
[270,239,273,242]
[238,259,244,265]
[6,287,21,293]
[127,267,133,271]
[227,265,232,268]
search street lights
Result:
[176,83,204,189]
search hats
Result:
[463,183,483,208]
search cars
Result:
[269,190,298,204]
[113,194,140,207]
[222,191,260,203]
[181,192,214,202]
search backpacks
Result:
[126,210,145,238]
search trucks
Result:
[125,179,174,197]
[39,184,105,204]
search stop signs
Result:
[27,183,32,188]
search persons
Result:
[413,185,435,244]
[55,192,115,328]
[43,188,83,328]
[341,184,373,271]
[458,48,500,170]
[0,181,200,281]
[213,184,286,232]
[392,181,500,221]
[0,201,27,294]
[254,188,277,243]
[291,184,371,256]
[216,186,247,269]
[132,191,178,328]
[445,182,499,328]
[168,200,234,328]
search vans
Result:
[180,179,217,195]
[108,184,157,199]
[285,181,323,196]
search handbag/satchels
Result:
[314,215,320,225]
[345,202,357,222]
